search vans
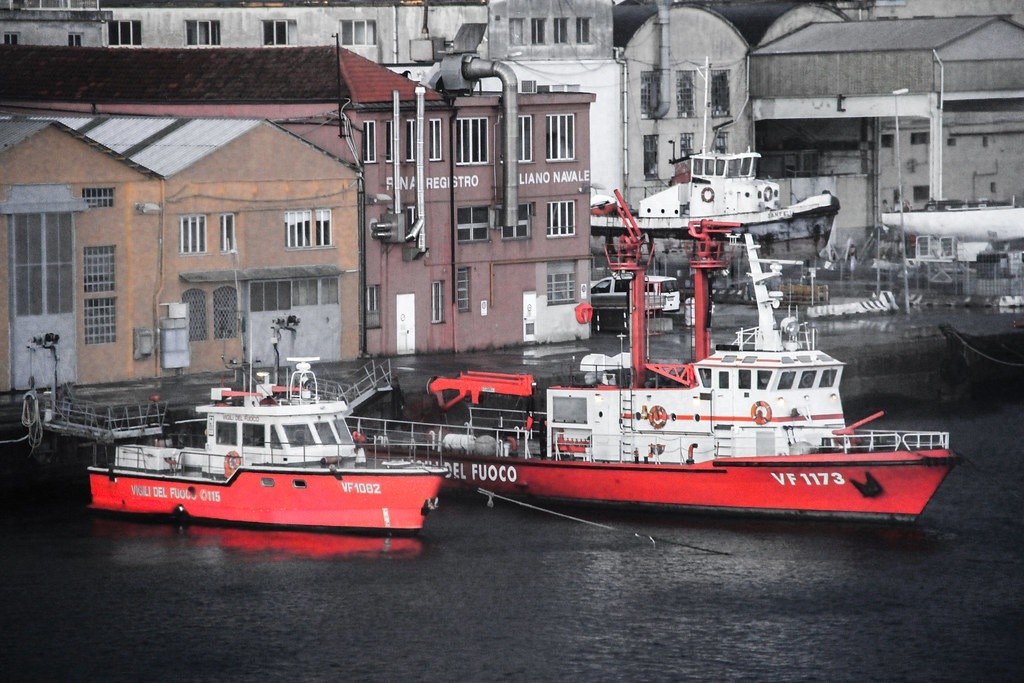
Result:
[589,276,681,310]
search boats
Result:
[352,233,963,527]
[937,319,1024,395]
[86,355,450,539]
[590,147,841,259]
[879,198,1024,246]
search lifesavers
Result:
[650,406,667,428]
[764,186,773,201]
[751,401,771,424]
[223,450,241,473]
[700,187,715,203]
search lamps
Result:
[135,202,162,216]
